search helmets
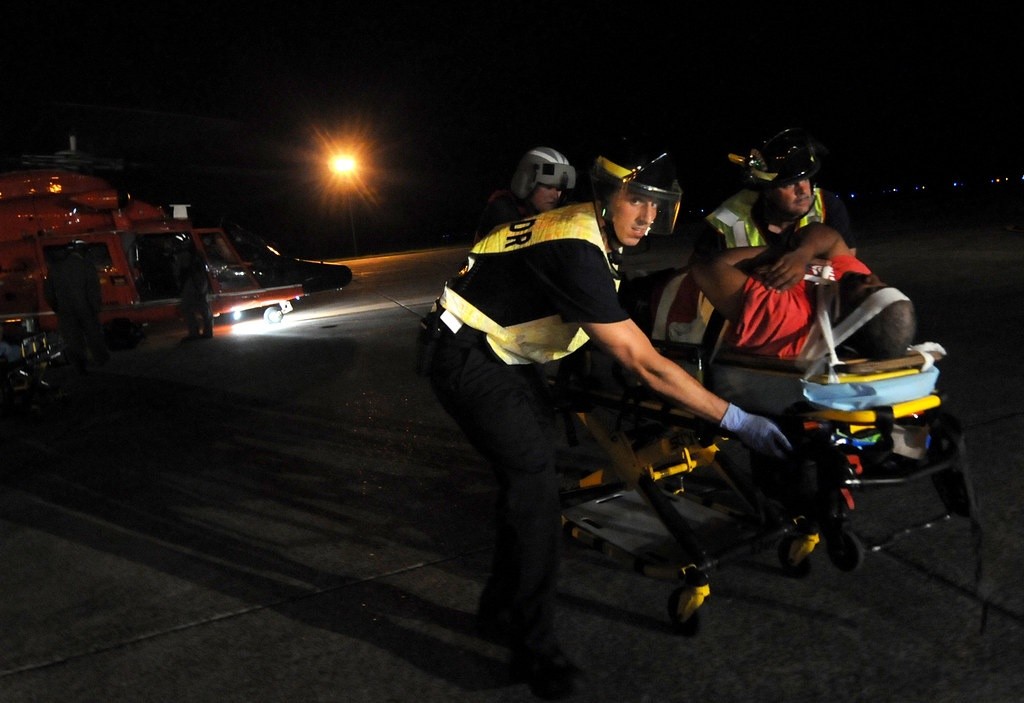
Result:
[588,137,683,202]
[67,239,88,249]
[175,231,191,248]
[510,146,576,199]
[746,125,822,191]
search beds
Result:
[535,341,979,633]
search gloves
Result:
[718,403,793,458]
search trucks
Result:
[0,169,305,354]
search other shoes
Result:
[182,334,200,342]
[478,572,570,621]
[515,634,591,700]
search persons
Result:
[55,238,113,373]
[425,136,796,693]
[469,143,920,365]
[179,239,215,340]
[690,122,857,520]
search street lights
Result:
[336,155,358,256]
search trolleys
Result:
[527,362,973,641]
